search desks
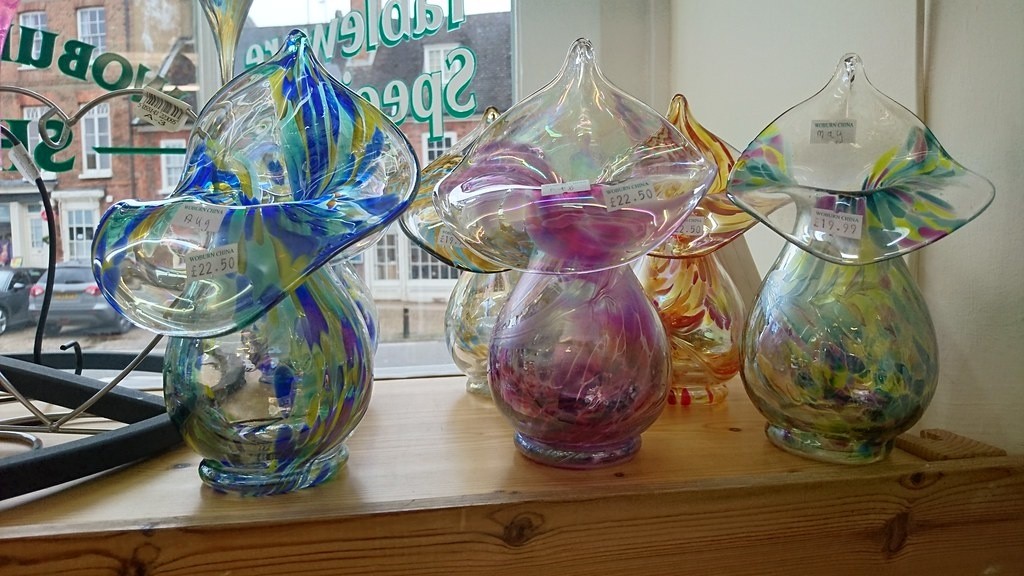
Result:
[0,374,1024,576]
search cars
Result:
[0,265,47,335]
[27,257,131,337]
